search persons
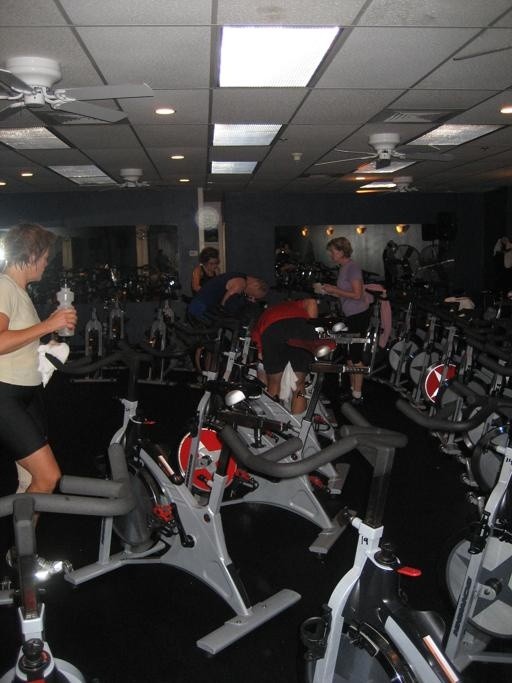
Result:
[500,236,511,301]
[188,271,269,327]
[153,249,170,273]
[311,236,370,404]
[250,297,321,414]
[382,240,408,293]
[491,238,512,296]
[192,246,222,294]
[1,222,79,570]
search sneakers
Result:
[340,391,366,401]
[351,395,367,410]
[9,550,64,577]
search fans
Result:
[86,166,166,194]
[316,132,466,200]
[0,53,152,128]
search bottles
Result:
[55,283,78,337]
